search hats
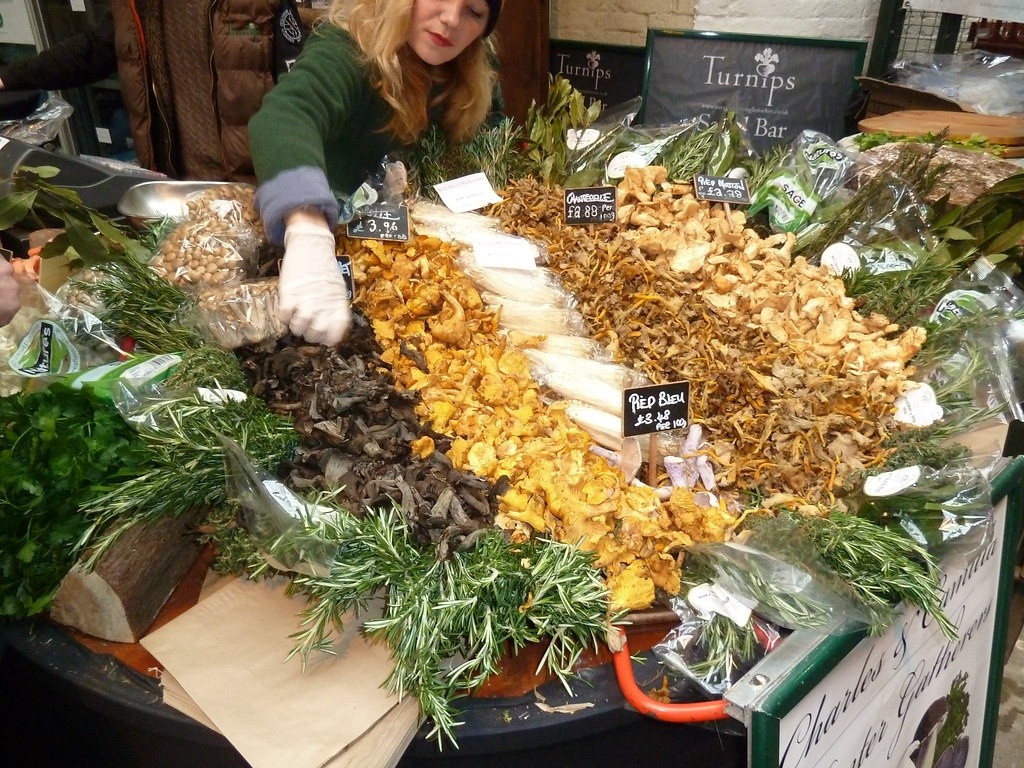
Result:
[484,0,505,38]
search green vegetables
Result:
[857,130,1005,157]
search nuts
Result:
[148,185,264,288]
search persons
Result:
[245,0,507,349]
[0,0,306,185]
